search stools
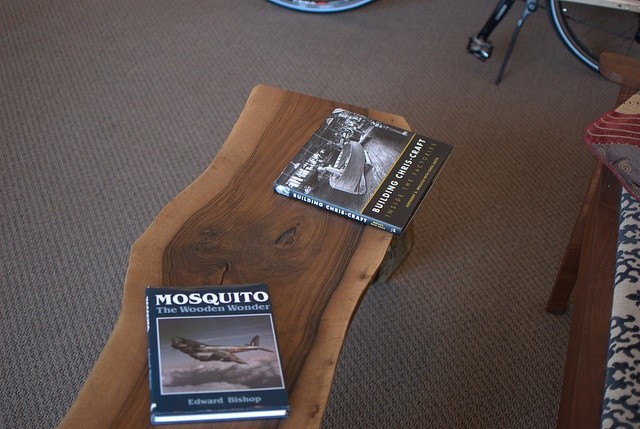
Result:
[50,81,418,429]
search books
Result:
[143,283,289,426]
[274,108,453,238]
